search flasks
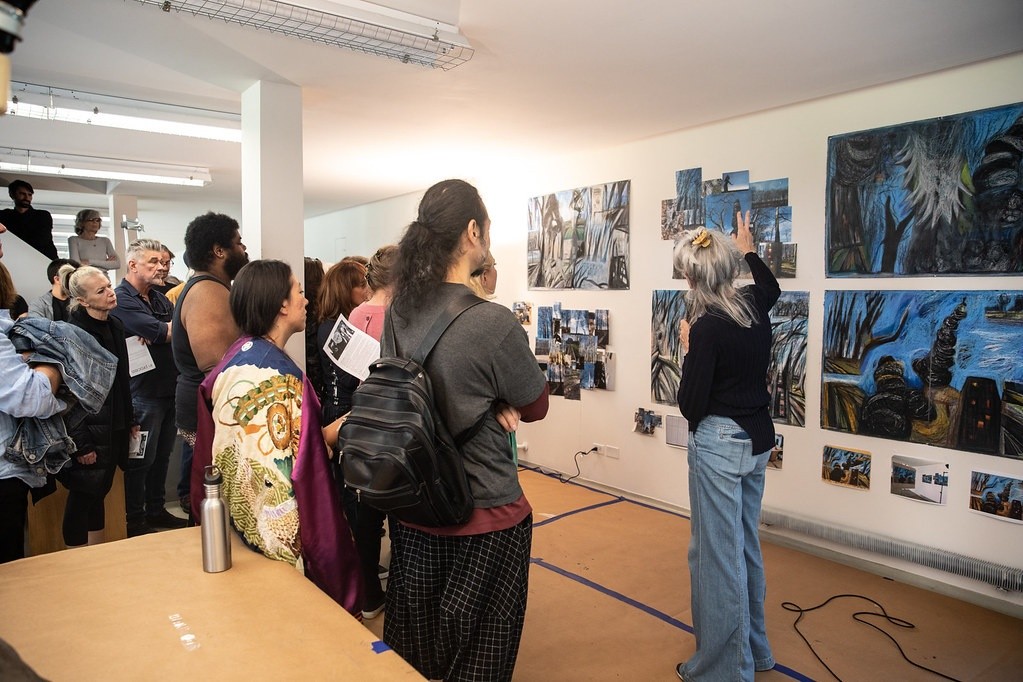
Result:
[201,465,232,573]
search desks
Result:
[0,522,428,682]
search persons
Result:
[172,207,250,449]
[0,223,62,563]
[673,210,782,682]
[380,179,549,682]
[191,260,366,625]
[68,209,121,282]
[9,239,195,548]
[469,249,518,469]
[585,312,608,336]
[0,179,59,261]
[304,246,400,619]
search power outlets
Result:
[591,443,604,455]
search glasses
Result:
[86,218,101,222]
[161,259,175,267]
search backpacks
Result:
[337,293,498,528]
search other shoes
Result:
[361,590,386,619]
[378,564,389,580]
[676,663,685,682]
[147,507,188,529]
